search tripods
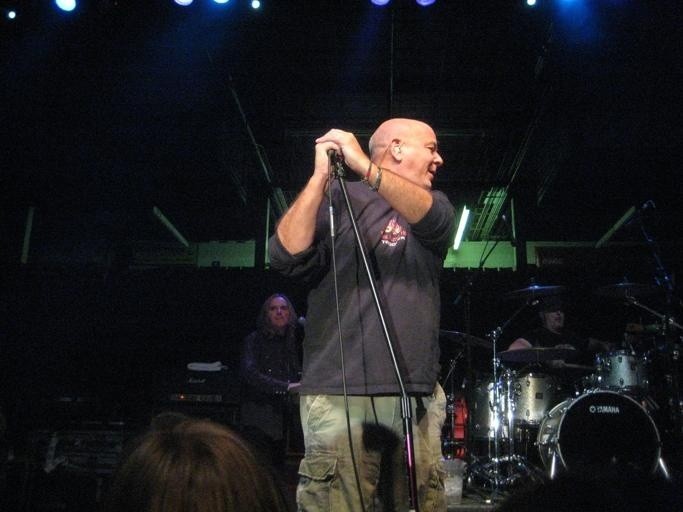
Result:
[465,348,547,504]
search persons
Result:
[233,292,304,468]
[505,296,614,402]
[265,115,460,512]
[98,411,291,512]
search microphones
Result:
[500,212,518,247]
[591,204,644,253]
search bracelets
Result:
[368,165,383,192]
[361,160,372,185]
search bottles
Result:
[595,352,612,385]
